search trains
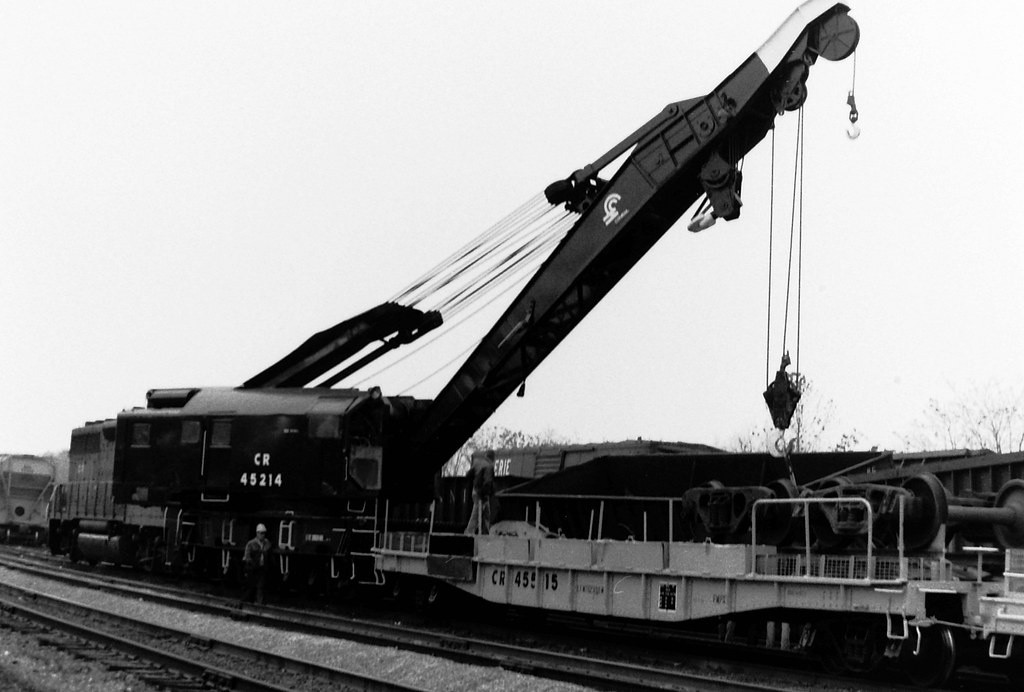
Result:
[0,0,1024,684]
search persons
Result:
[243,523,272,607]
[464,451,498,535]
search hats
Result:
[256,523,267,532]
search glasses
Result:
[259,532,266,534]
